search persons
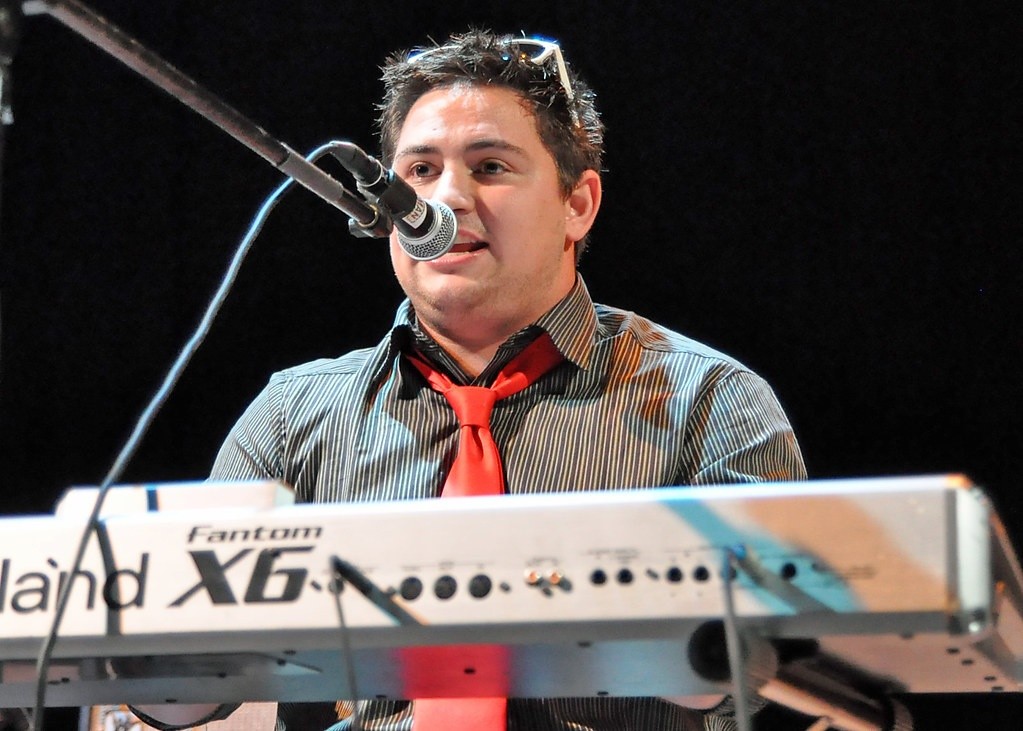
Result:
[127,25,810,731]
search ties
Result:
[405,332,562,731]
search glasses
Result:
[406,38,584,133]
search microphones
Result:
[328,140,458,262]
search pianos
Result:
[0,464,1021,715]
